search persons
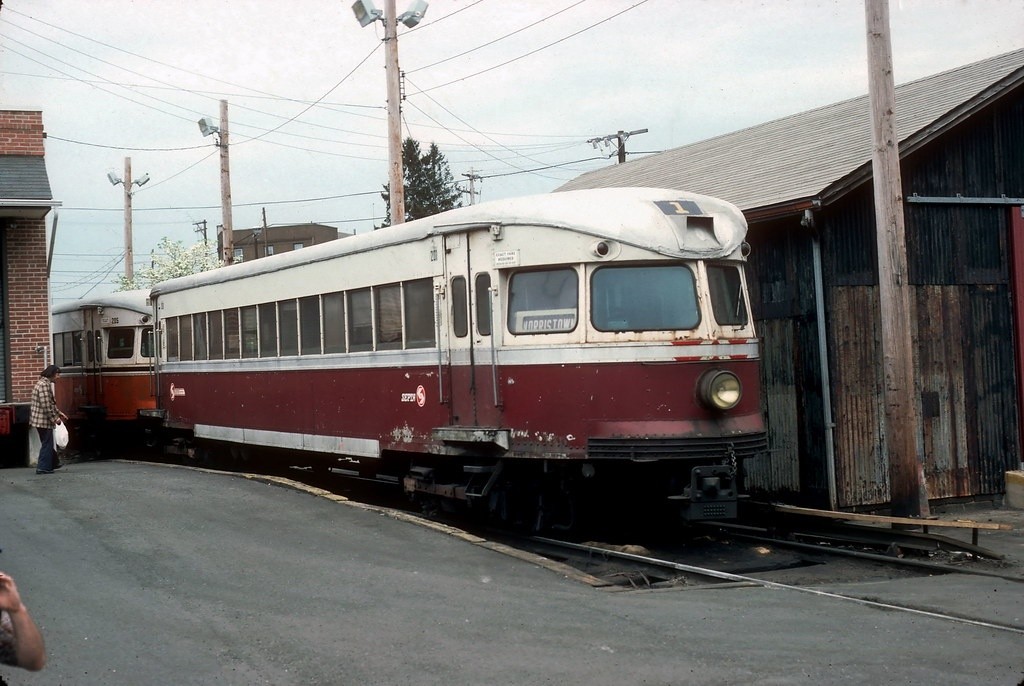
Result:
[30,364,70,474]
[0,572,45,685]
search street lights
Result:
[107,157,150,281]
[352,0,429,227]
[198,99,234,266]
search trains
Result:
[52,187,768,536]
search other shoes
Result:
[36,468,55,474]
[53,464,62,469]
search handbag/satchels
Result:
[54,420,69,450]
